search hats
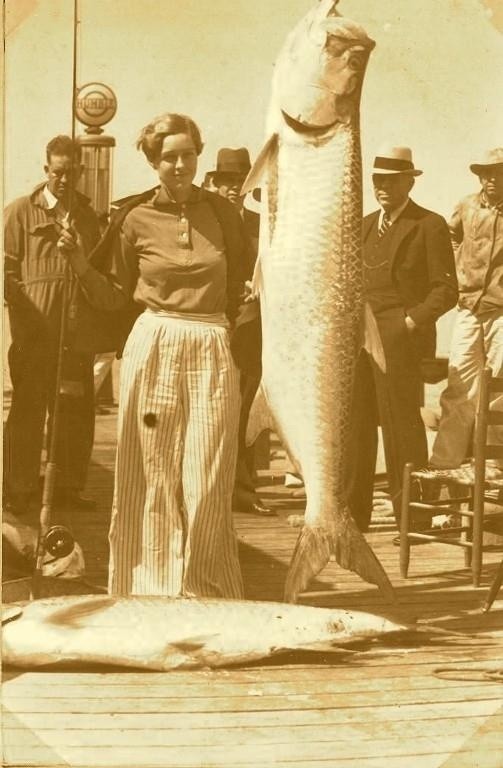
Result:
[207,147,251,175]
[470,147,503,175]
[372,147,423,176]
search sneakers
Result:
[392,533,429,545]
[430,515,459,537]
[3,492,31,514]
[52,489,97,510]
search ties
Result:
[55,200,68,237]
[378,212,392,238]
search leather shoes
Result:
[249,499,276,515]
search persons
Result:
[427,146,503,470]
[201,171,218,195]
[212,148,277,518]
[55,110,254,603]
[2,135,104,518]
[346,146,460,547]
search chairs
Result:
[397,366,503,588]
